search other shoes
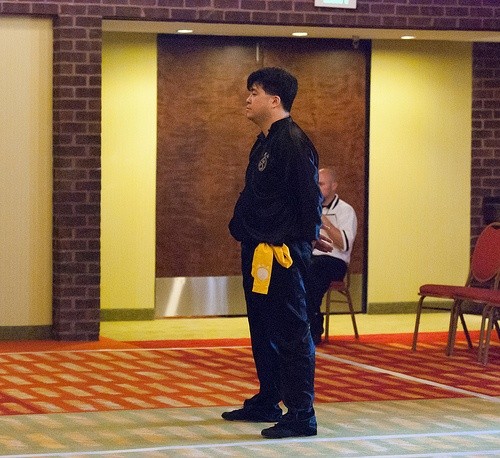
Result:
[221,407,282,422]
[261,421,317,439]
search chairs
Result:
[411,222,500,367]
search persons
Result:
[220,68,333,439]
[306,167,357,344]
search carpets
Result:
[0,327,500,415]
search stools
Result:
[325,265,359,343]
